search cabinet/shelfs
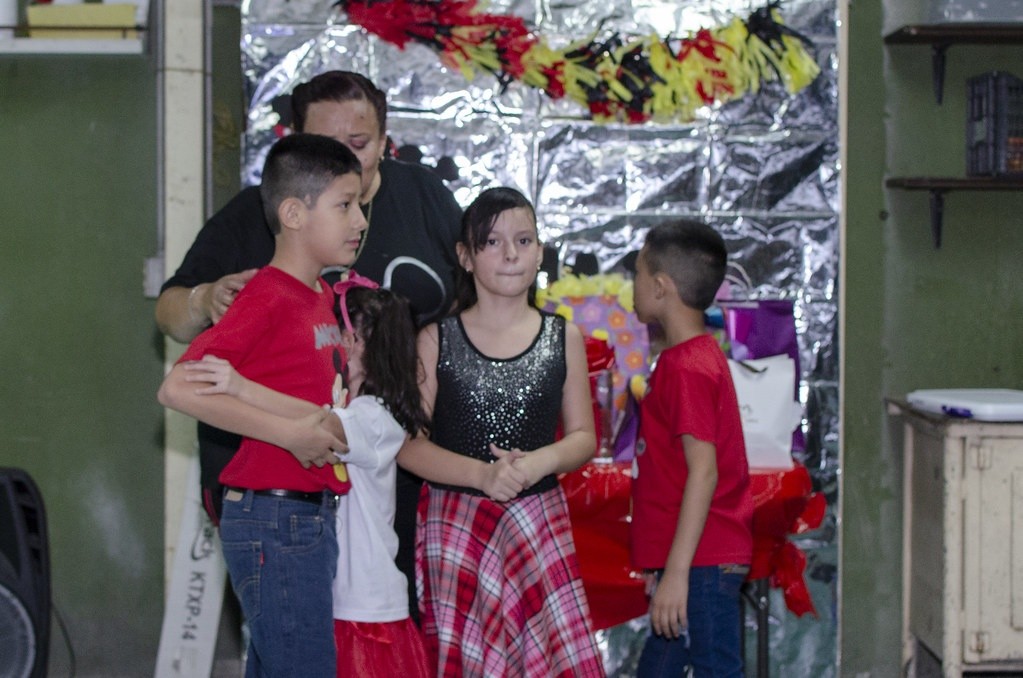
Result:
[882,21,1023,252]
[885,395,1023,678]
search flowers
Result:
[536,271,652,417]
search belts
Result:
[228,487,340,509]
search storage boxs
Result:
[25,2,137,38]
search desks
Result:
[559,460,829,678]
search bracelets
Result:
[187,284,212,329]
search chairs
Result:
[0,465,52,678]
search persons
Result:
[629,217,754,678]
[154,69,606,678]
